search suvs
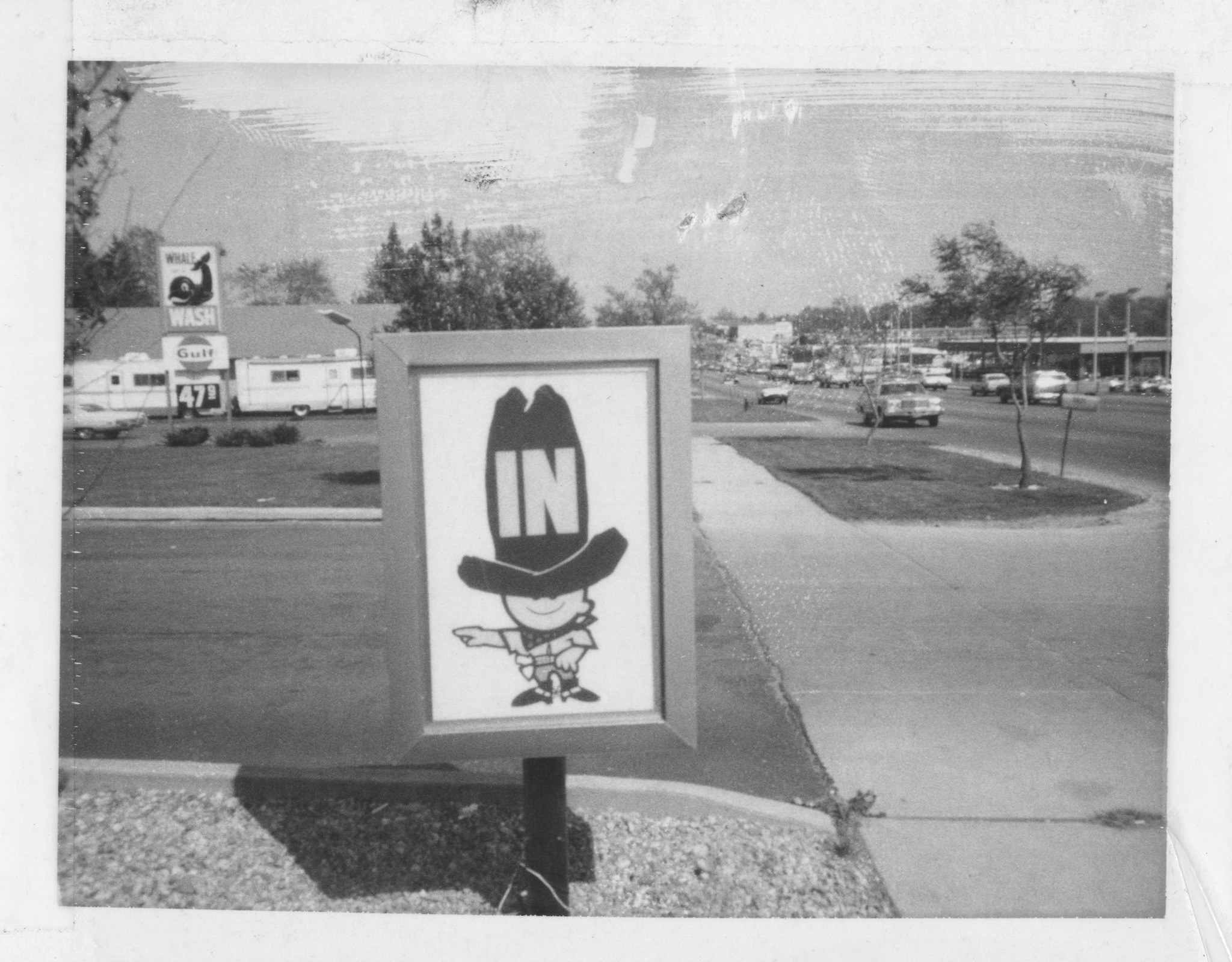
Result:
[1007,369,1073,408]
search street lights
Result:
[1123,287,1143,392]
[1163,283,1172,379]
[318,309,366,416]
[1090,290,1107,394]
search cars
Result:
[1076,373,1172,396]
[696,344,952,407]
[970,373,1011,397]
[859,376,945,429]
[63,394,148,442]
